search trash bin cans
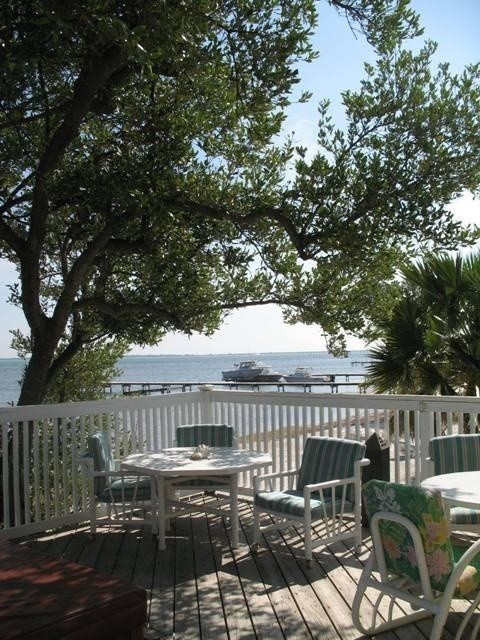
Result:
[361,433,390,526]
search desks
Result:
[0,540,147,640]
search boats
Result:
[221,359,283,385]
[281,367,331,384]
